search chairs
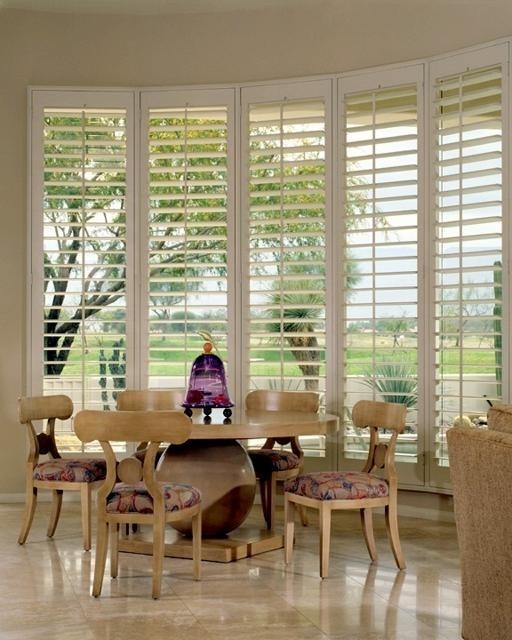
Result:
[443,424,512,640]
[68,407,206,603]
[17,393,119,554]
[281,397,409,580]
[114,387,206,538]
[242,386,322,533]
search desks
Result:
[114,407,341,565]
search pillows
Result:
[482,396,512,434]
